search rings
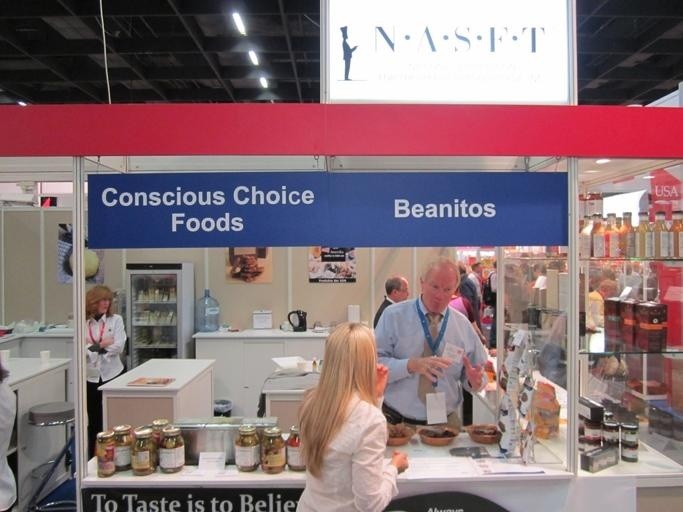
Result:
[427,366,433,371]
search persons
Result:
[296,320,408,512]
[373,255,489,435]
[374,275,409,330]
[448,252,658,388]
[0,352,17,512]
[86,285,127,462]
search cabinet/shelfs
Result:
[190,324,374,426]
[0,356,71,511]
[0,329,73,359]
[261,377,314,434]
[94,358,216,433]
[496,158,679,475]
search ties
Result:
[417,314,440,406]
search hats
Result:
[85,285,114,307]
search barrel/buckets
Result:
[619,298,647,345]
[193,288,221,332]
[603,296,629,338]
[634,301,668,352]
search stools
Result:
[25,402,77,511]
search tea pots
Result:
[287,309,308,332]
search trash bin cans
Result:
[214,399,232,417]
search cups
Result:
[38,350,50,364]
[313,320,325,333]
[0,349,11,367]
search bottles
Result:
[579,210,682,260]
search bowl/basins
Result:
[419,426,459,445]
[467,424,504,446]
[386,425,415,447]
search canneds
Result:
[261,427,286,474]
[579,413,639,463]
[286,425,306,472]
[234,425,260,472]
[96,419,185,477]
